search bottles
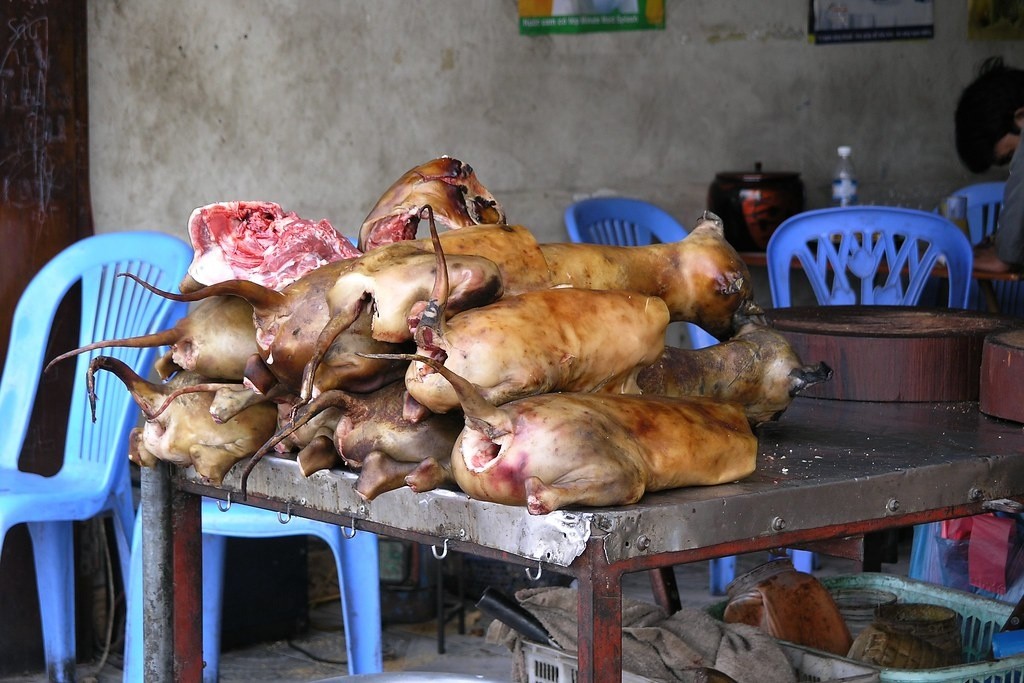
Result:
[831,146,858,209]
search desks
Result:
[147,386,1023,683]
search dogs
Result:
[39,153,835,520]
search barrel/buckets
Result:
[707,161,805,252]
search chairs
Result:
[768,206,981,575]
[0,230,223,681]
[939,182,1021,316]
[563,191,818,595]
[120,499,384,678]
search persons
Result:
[952,60,1024,277]
